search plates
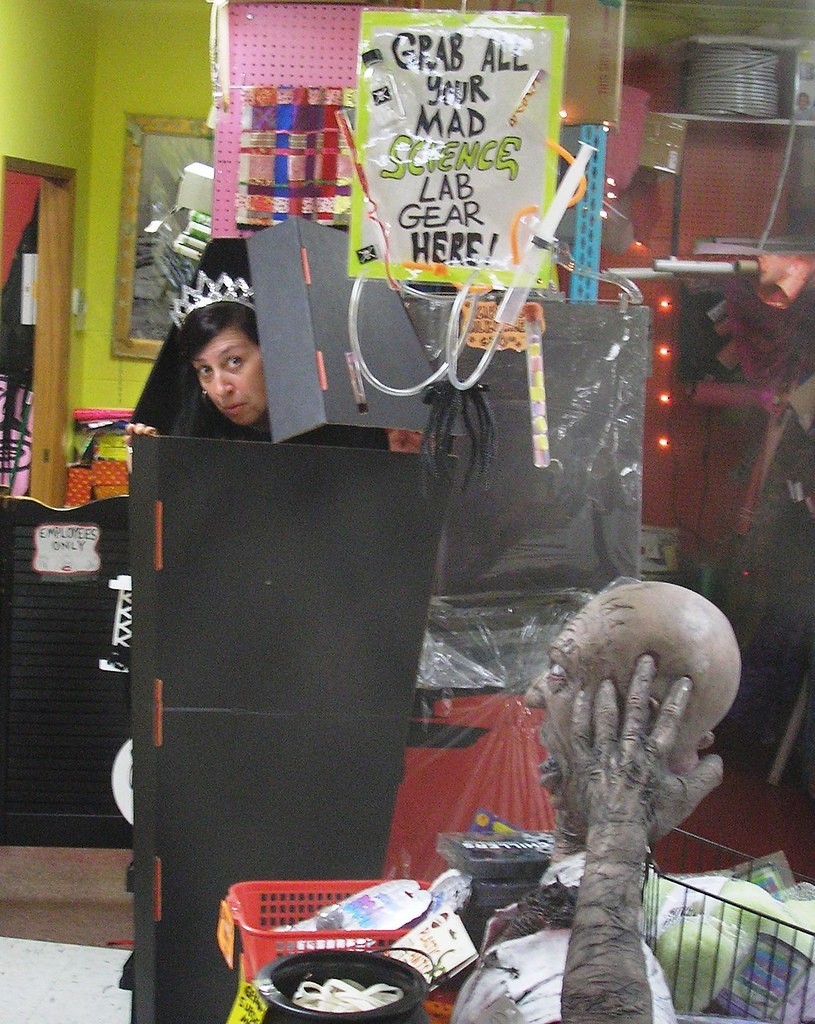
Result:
[682,47,780,121]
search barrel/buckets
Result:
[255,948,434,1024]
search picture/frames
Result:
[113,113,218,361]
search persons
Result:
[448,582,742,1024]
[124,270,390,452]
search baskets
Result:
[225,879,433,981]
[642,828,815,1024]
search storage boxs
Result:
[421,0,625,127]
[64,434,130,507]
